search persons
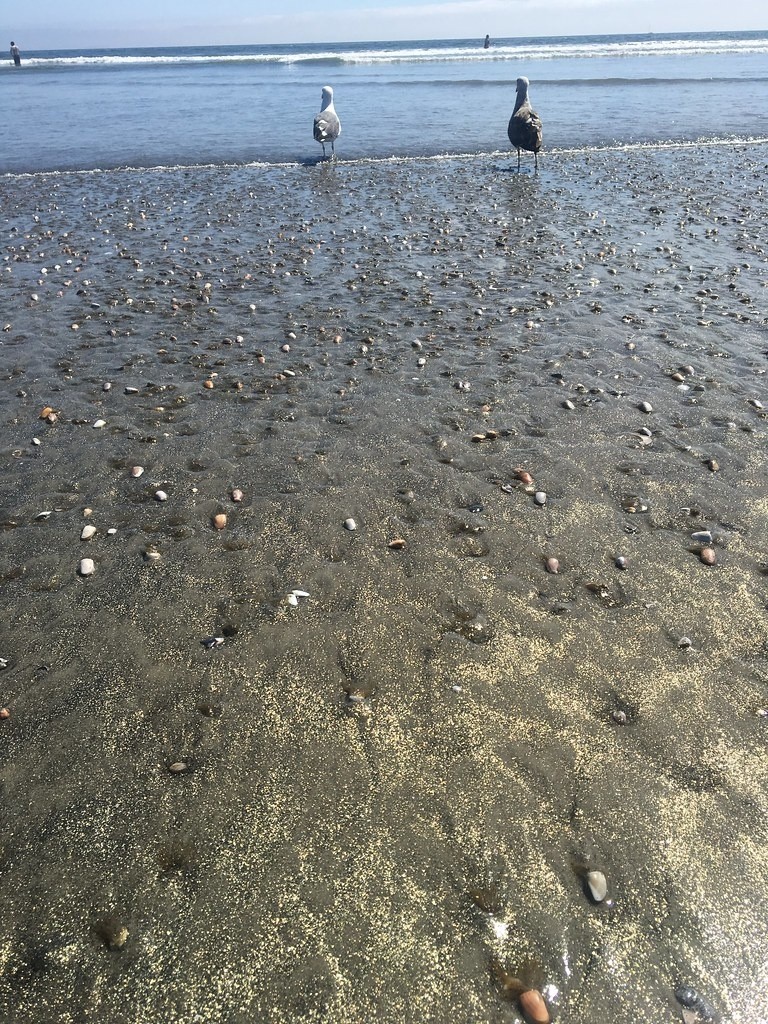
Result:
[484,35,490,48]
[9,41,21,66]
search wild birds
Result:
[312,85,341,158]
[507,76,542,170]
